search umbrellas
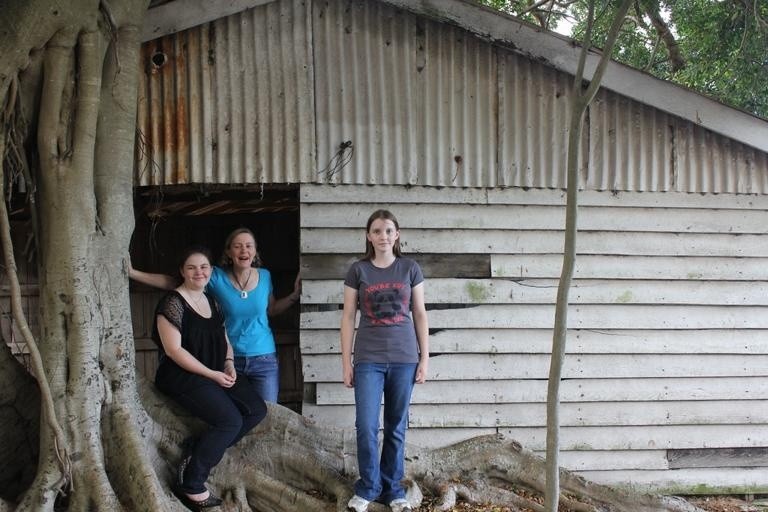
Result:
[184,491,224,507]
[177,438,189,486]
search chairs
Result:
[389,498,413,512]
[347,494,369,512]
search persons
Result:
[151,246,268,509]
[121,226,301,407]
[338,210,431,512]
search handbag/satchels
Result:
[287,294,296,304]
[224,358,234,362]
